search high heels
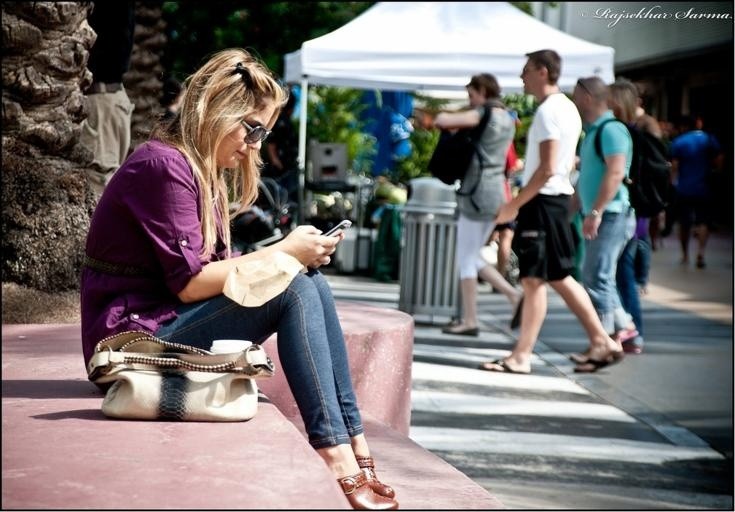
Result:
[337,468,400,512]
[356,452,398,500]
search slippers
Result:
[573,349,625,373]
[477,356,533,376]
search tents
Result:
[285,2,614,226]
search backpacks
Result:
[575,118,675,217]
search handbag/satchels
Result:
[83,328,278,425]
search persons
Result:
[607,80,672,355]
[81,50,398,511]
[481,50,622,375]
[82,0,134,193]
[572,78,676,362]
[673,114,718,268]
[435,74,525,336]
[265,93,299,207]
[482,110,525,293]
[635,96,678,251]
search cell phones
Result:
[323,220,352,237]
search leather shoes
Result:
[511,292,525,330]
[441,325,479,338]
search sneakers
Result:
[622,342,646,357]
[695,260,708,269]
[610,324,640,345]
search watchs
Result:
[590,209,601,216]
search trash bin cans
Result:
[397,176,461,323]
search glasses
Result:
[240,119,274,145]
